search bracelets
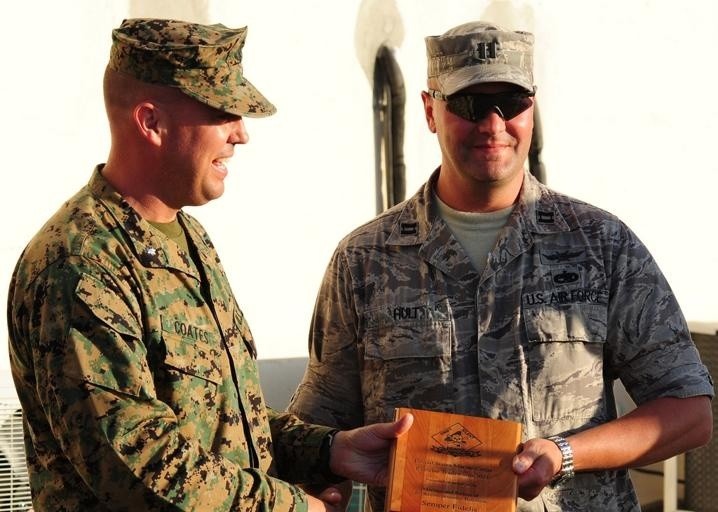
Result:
[323,430,337,472]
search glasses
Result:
[424,86,533,120]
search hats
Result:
[110,18,276,117]
[424,21,534,97]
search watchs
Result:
[546,433,576,491]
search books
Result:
[384,406,525,512]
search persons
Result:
[278,23,713,512]
[6,16,414,512]
